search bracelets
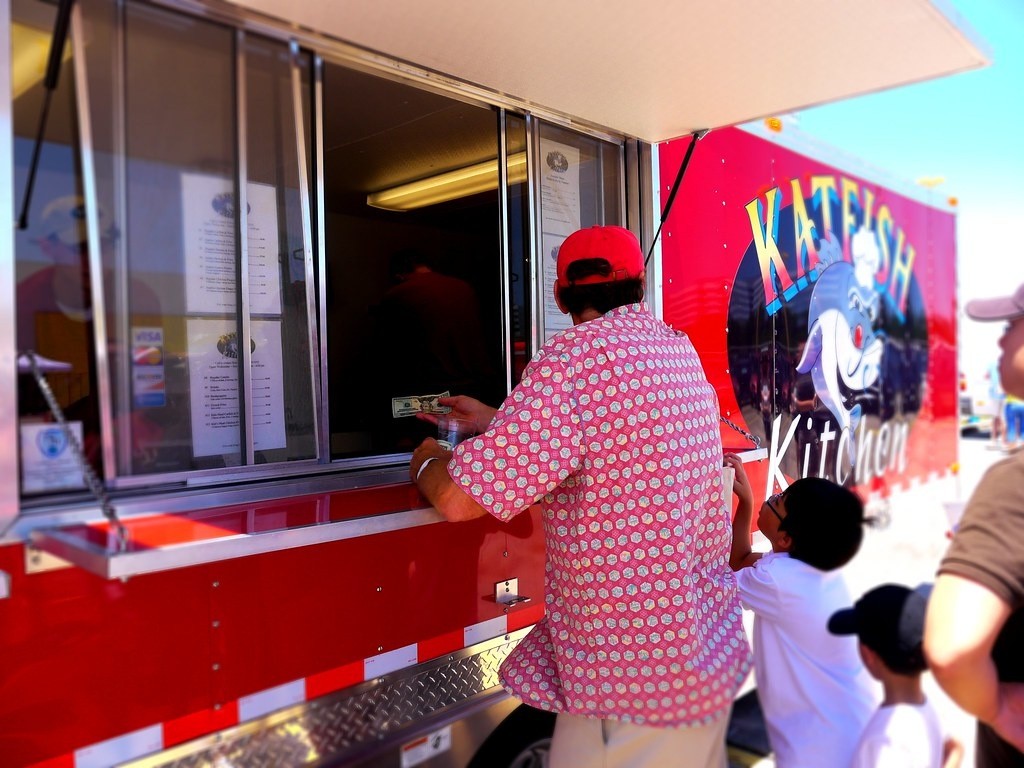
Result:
[416,457,437,483]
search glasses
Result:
[767,493,784,523]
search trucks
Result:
[1,1,991,768]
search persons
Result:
[923,285,1024,768]
[410,224,752,768]
[723,452,880,768]
[16,196,175,479]
[827,585,965,768]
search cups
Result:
[437,418,477,451]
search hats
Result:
[40,197,112,244]
[557,224,645,287]
[966,283,1024,320]
[828,584,926,653]
